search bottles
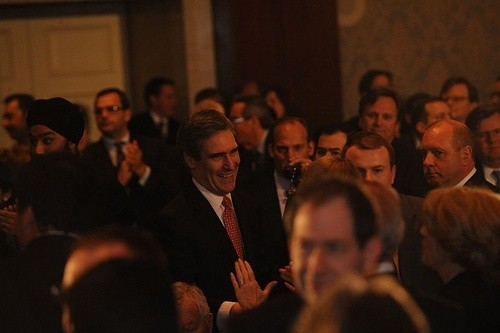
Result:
[285,165,300,215]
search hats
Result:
[26,97,85,146]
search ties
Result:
[221,194,243,263]
[112,142,124,176]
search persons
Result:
[0,71,500,333]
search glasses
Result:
[229,114,252,125]
[94,105,124,115]
